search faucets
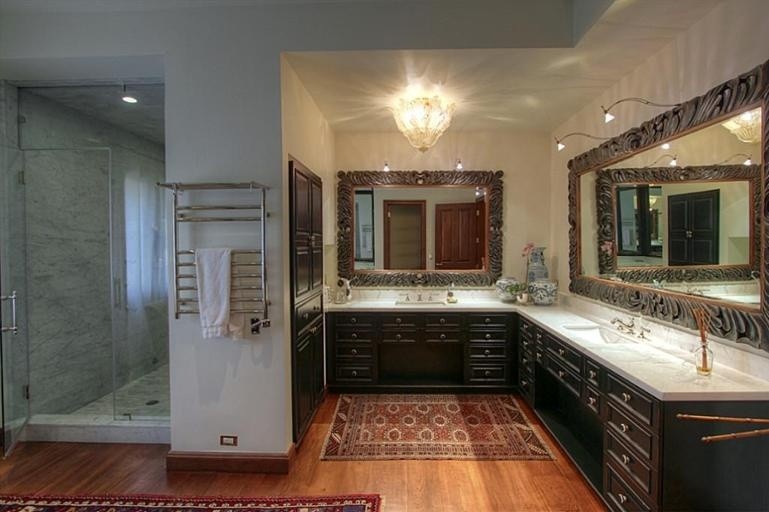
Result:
[610,316,636,335]
[686,288,704,296]
[416,287,423,301]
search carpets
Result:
[1,494,381,511]
[318,393,554,461]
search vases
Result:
[496,247,559,306]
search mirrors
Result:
[336,168,505,289]
[568,61,769,356]
[593,165,761,281]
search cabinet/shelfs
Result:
[326,312,516,391]
[516,313,768,512]
[288,158,325,448]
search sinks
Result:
[394,301,446,308]
[562,324,639,347]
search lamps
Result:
[648,151,755,169]
[555,96,680,154]
[391,96,455,152]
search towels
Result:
[194,247,245,338]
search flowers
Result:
[504,242,534,294]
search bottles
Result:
[695,341,712,375]
